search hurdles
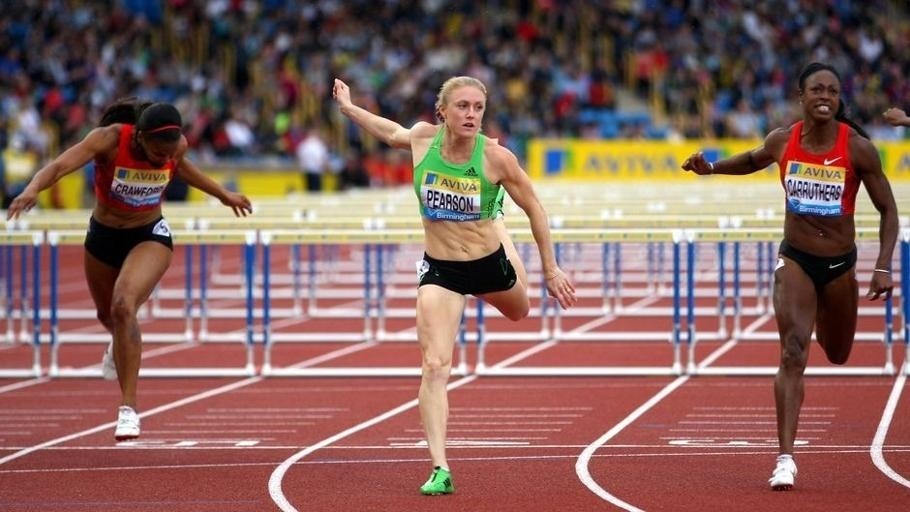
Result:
[0,206,113,344]
[475,227,683,376]
[0,228,43,378]
[140,184,424,344]
[375,216,551,341]
[44,230,257,377]
[456,181,910,316]
[728,215,909,342]
[684,226,905,376]
[551,215,732,342]
[258,229,471,377]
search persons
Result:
[7,102,252,441]
[332,78,579,496]
[681,62,900,489]
[0,0,909,190]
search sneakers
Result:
[491,183,506,219]
[768,453,797,488]
[420,466,455,494]
[115,406,141,439]
[102,341,119,379]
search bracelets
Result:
[544,271,559,280]
[875,269,890,274]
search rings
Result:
[567,288,572,293]
